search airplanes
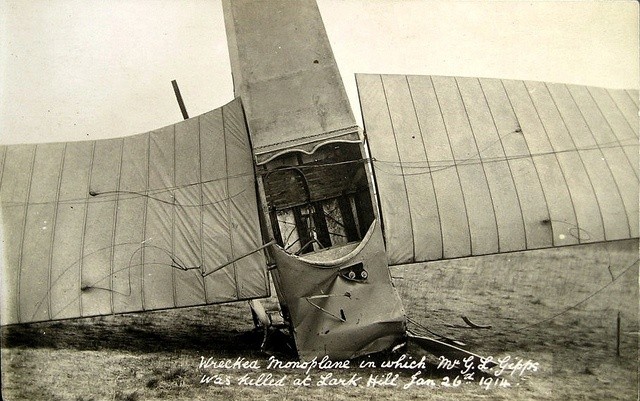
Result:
[0,0,640,373]
